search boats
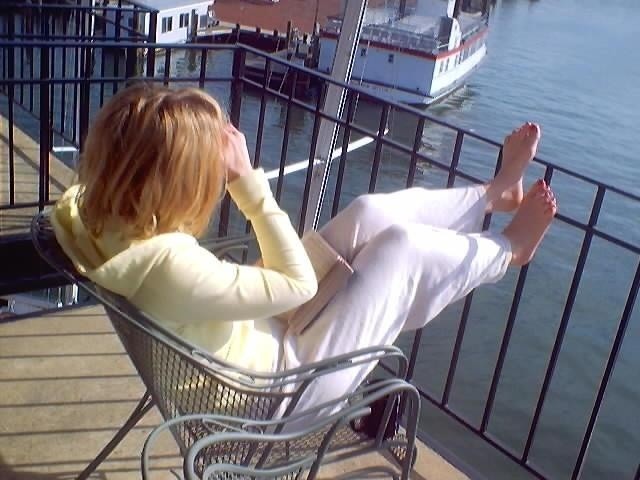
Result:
[316,0,492,105]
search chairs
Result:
[139,379,422,480]
[30,210,407,479]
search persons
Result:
[48,83,559,434]
